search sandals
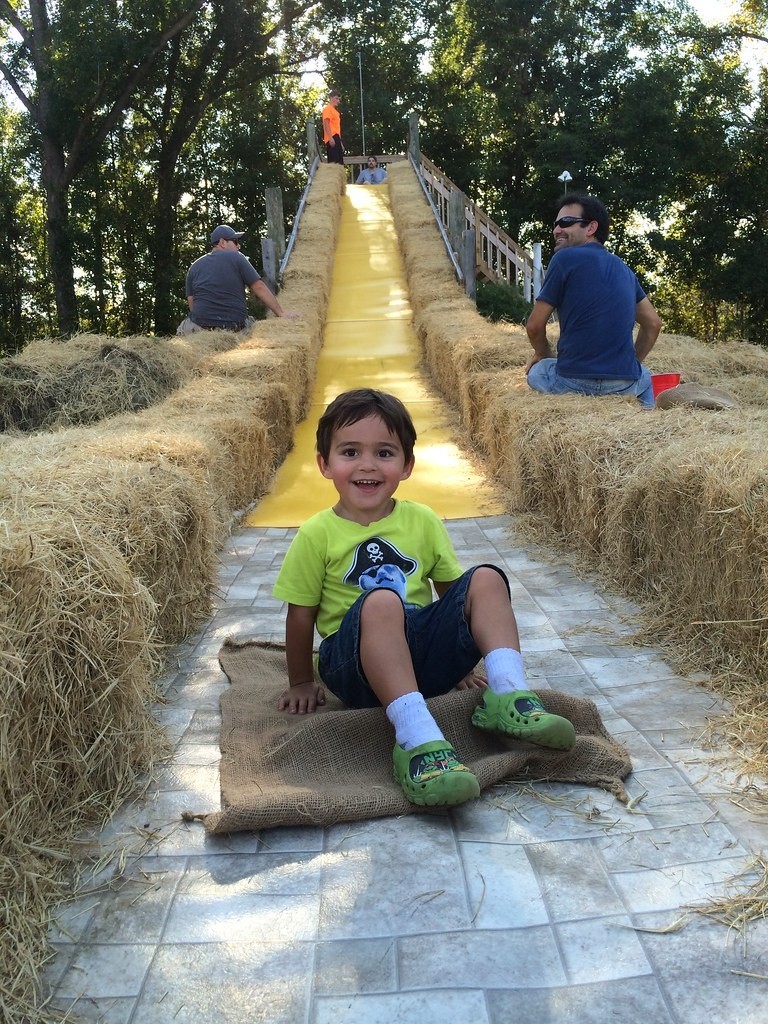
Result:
[393,742,480,805]
[471,686,575,751]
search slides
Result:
[254,180,521,526]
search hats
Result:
[211,225,245,242]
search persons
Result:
[176,223,299,336]
[276,389,587,809]
[355,157,388,186]
[524,194,663,411]
[322,89,345,166]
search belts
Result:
[202,326,244,333]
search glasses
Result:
[224,238,237,246]
[554,216,588,228]
